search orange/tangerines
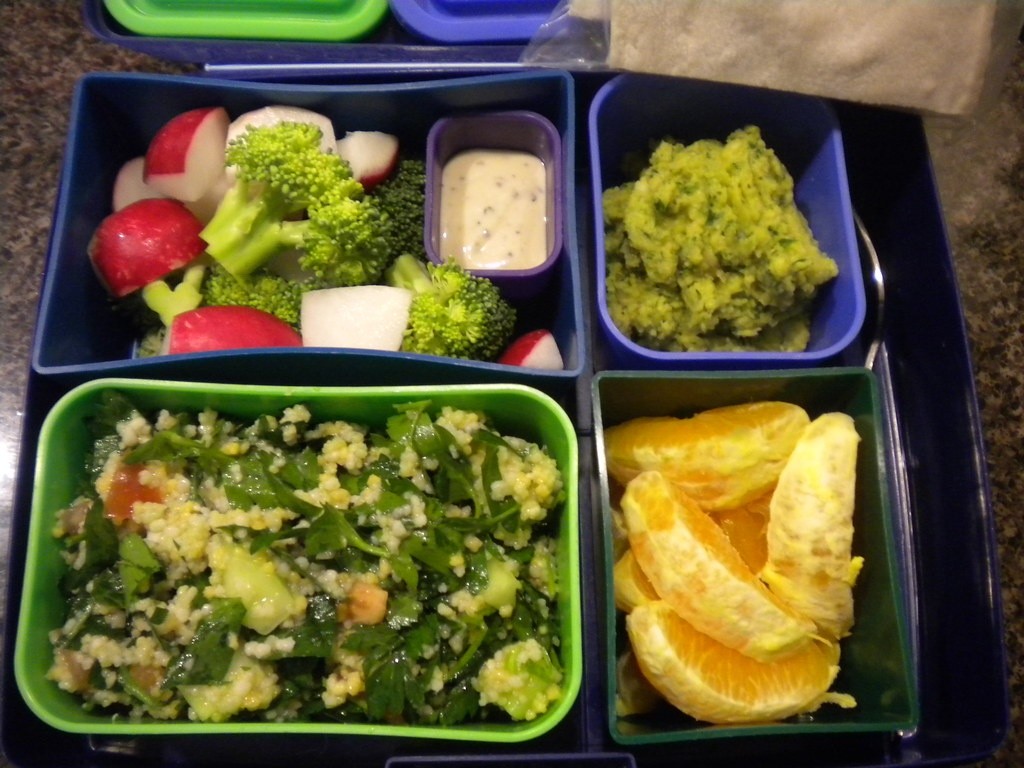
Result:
[600,399,861,726]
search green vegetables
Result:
[51,386,559,728]
[140,117,519,364]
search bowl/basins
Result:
[12,382,579,742]
[589,76,867,360]
[422,110,562,275]
[106,0,572,41]
[589,369,920,744]
[41,67,585,381]
